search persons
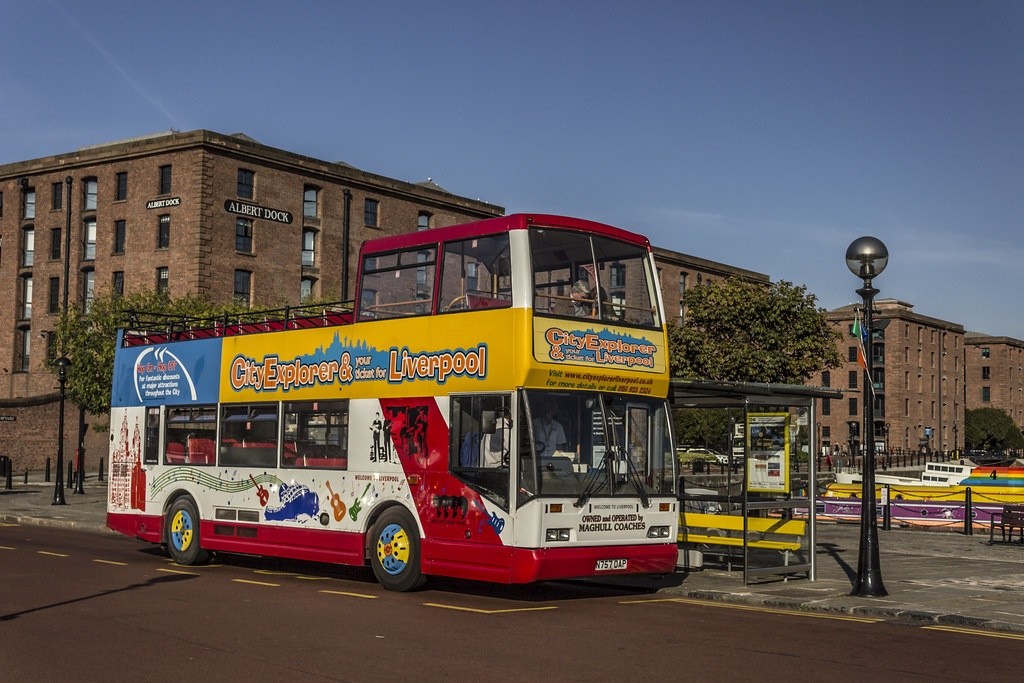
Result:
[479,405,511,468]
[531,395,567,457]
[553,280,593,319]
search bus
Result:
[104,212,683,593]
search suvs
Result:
[687,448,729,465]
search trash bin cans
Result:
[693,460,704,472]
[0,455,9,477]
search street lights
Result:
[883,423,890,465]
[52,357,75,505]
[951,420,960,461]
[816,421,822,471]
[850,422,857,468]
[846,235,890,599]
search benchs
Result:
[124,302,377,348]
[166,434,347,468]
[676,512,806,583]
[444,289,512,310]
[990,505,1024,545]
